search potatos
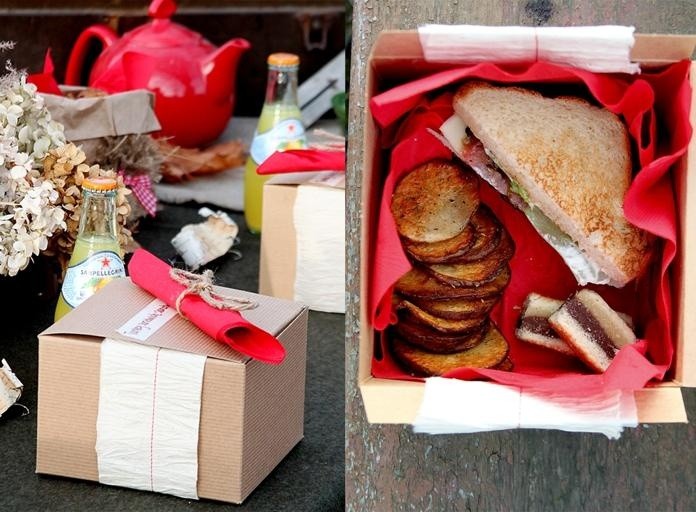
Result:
[384,158,517,379]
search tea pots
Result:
[66,0,251,148]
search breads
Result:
[515,287,639,371]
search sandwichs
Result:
[441,82,661,287]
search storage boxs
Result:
[353,29,696,440]
[32,278,307,507]
[260,129,348,316]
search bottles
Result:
[53,177,126,324]
[243,53,308,234]
